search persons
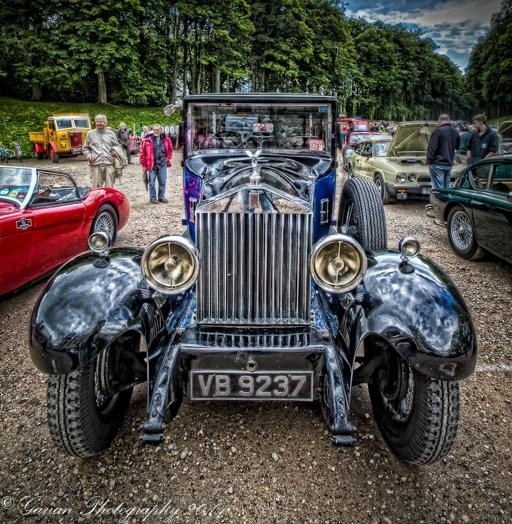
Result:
[139,124,173,204]
[370,120,402,136]
[441,117,477,135]
[464,113,499,171]
[82,115,124,190]
[426,113,460,219]
[115,122,153,164]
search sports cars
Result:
[0,163,130,297]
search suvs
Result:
[27,93,476,467]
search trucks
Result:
[27,110,94,162]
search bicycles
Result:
[1,140,23,162]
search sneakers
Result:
[150,199,168,204]
[426,210,446,227]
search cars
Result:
[429,154,511,268]
[336,115,512,204]
